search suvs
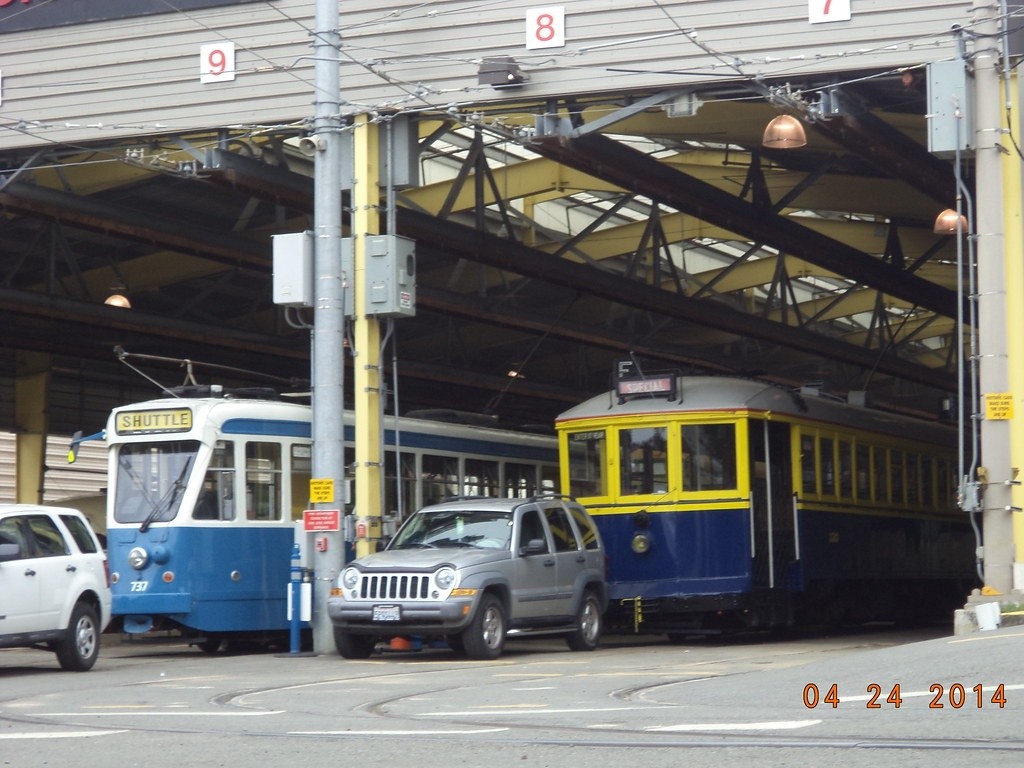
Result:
[326,493,607,660]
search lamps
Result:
[933,208,968,233]
[104,294,131,308]
[479,54,523,90]
[762,114,807,147]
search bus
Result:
[554,359,980,633]
[104,352,599,653]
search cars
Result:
[0,504,112,671]
